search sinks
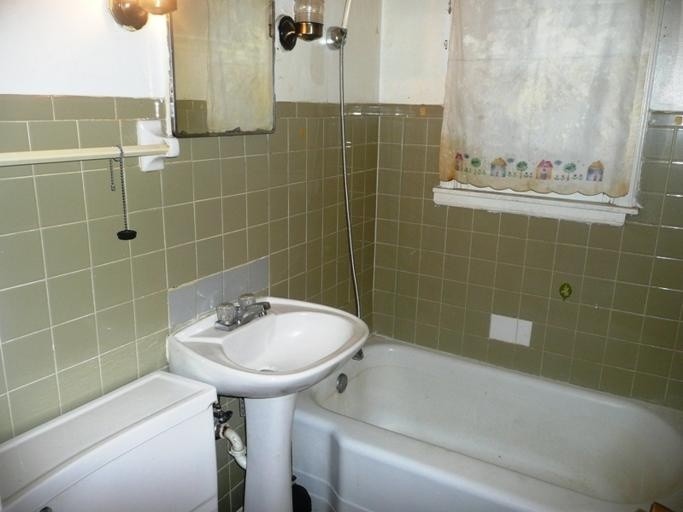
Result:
[167,296,371,397]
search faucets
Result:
[216,293,269,330]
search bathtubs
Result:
[291,333,683,508]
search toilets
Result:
[0,371,217,507]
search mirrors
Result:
[166,0,275,138]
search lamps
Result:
[109,0,177,32]
[278,0,324,50]
